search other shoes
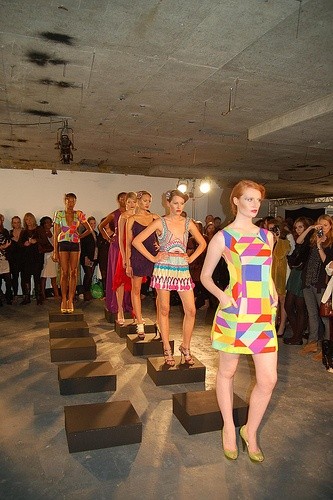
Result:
[283,336,303,345]
[0,289,62,305]
[296,340,318,354]
[303,332,310,339]
[313,351,322,361]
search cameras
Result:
[273,225,279,232]
[317,228,323,238]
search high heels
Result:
[178,343,195,365]
[136,323,145,340]
[239,425,264,463]
[60,300,74,314]
[222,425,238,460]
[118,310,124,325]
[162,344,175,366]
[275,323,286,338]
[132,310,145,324]
[153,323,162,341]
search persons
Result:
[125,190,161,339]
[97,192,133,313]
[199,180,279,462]
[51,193,92,313]
[112,191,145,325]
[0,211,333,373]
[132,190,207,365]
[17,212,44,306]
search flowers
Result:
[137,192,142,199]
[165,191,172,199]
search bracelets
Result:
[317,247,322,250]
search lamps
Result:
[177,176,189,193]
[200,176,211,193]
[54,120,74,165]
